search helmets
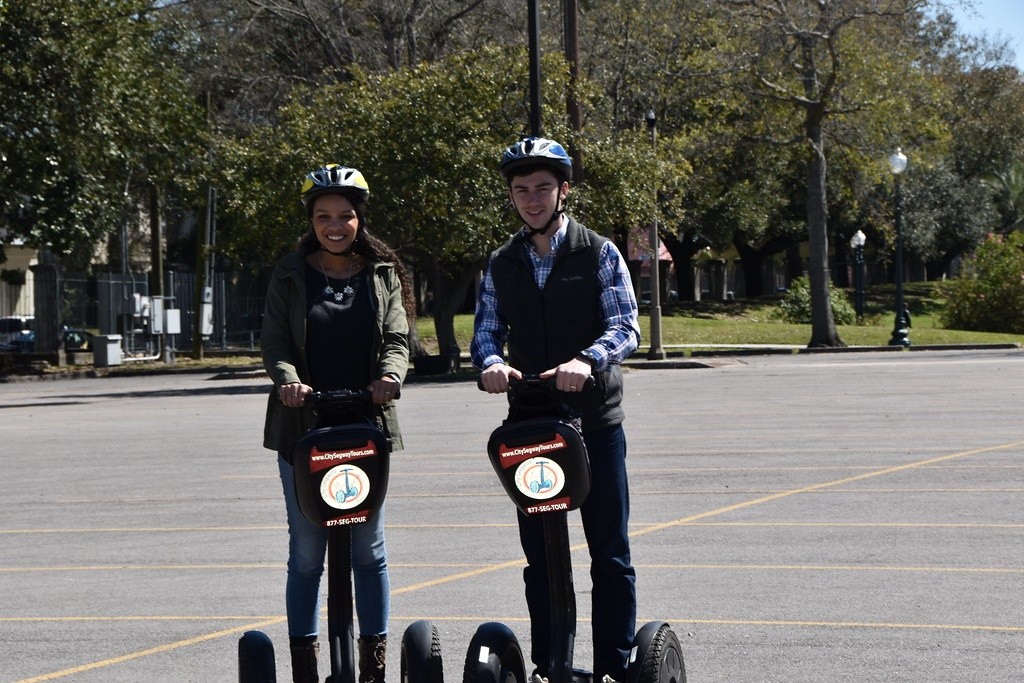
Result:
[300,163,370,207]
[498,137,571,183]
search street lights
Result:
[849,229,866,327]
[887,149,911,346]
[642,108,669,356]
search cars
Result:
[640,290,680,310]
[1,331,98,360]
[0,315,81,346]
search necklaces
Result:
[319,251,353,302]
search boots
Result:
[357,633,387,683]
[289,633,319,683]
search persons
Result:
[259,162,414,683]
[469,137,641,683]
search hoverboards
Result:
[236,387,445,683]
[462,372,687,683]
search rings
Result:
[386,392,391,394]
[570,386,576,390]
